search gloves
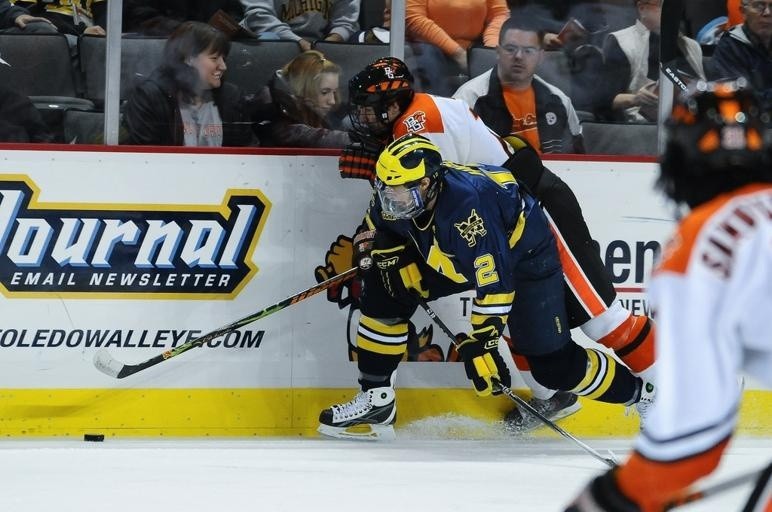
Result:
[368,236,435,307]
[456,327,511,397]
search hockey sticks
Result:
[419,299,621,468]
[93,265,359,378]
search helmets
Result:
[347,55,412,136]
[657,75,767,201]
[372,133,441,220]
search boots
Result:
[319,387,397,426]
[503,392,578,431]
[635,379,657,439]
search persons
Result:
[350,58,655,428]
[562,94,772,510]
[320,146,654,428]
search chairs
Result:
[0,0,713,160]
